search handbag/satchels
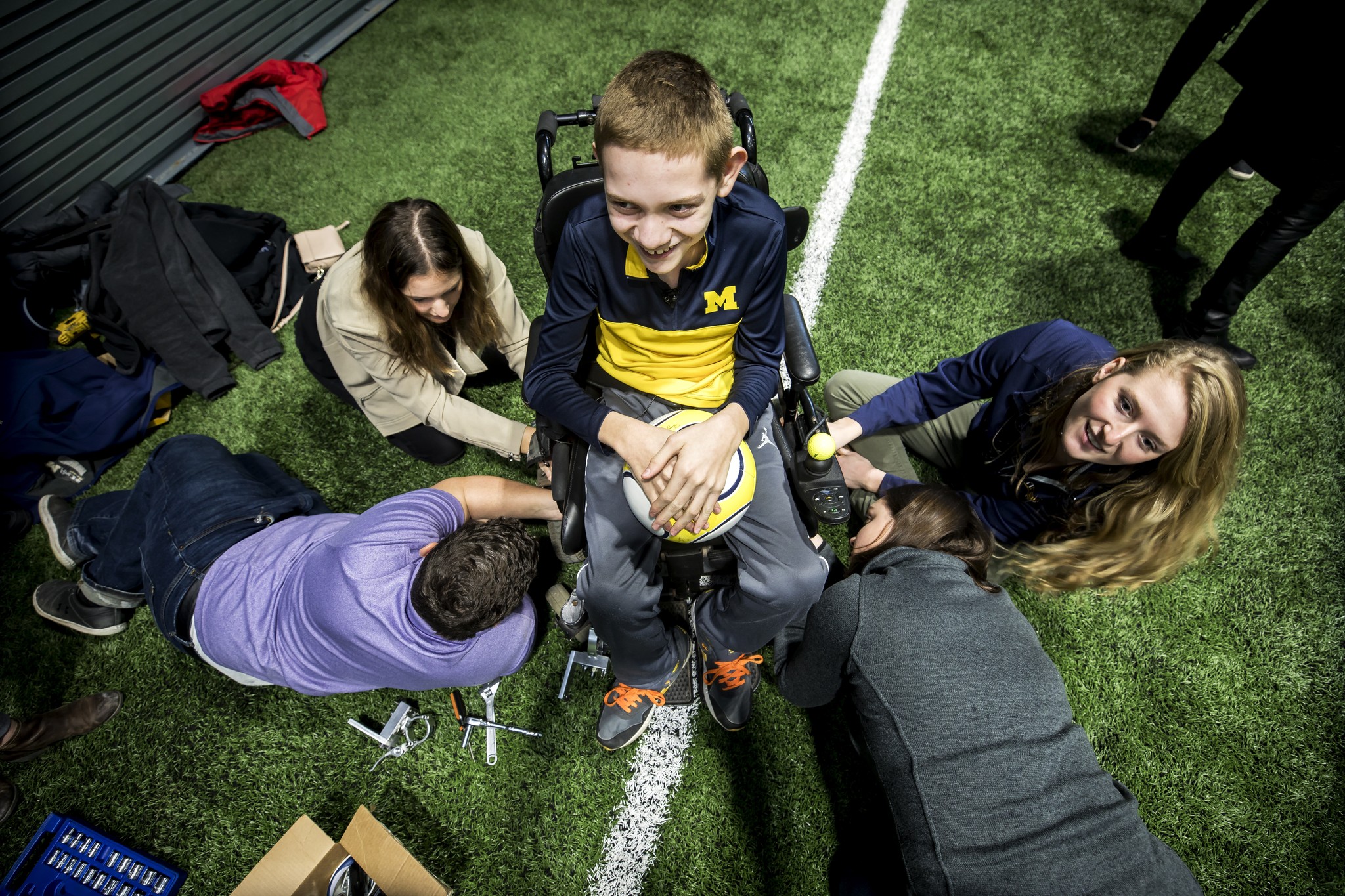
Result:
[289,217,354,278]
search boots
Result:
[1120,218,1178,261]
[1191,307,1259,368]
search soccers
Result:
[621,409,756,545]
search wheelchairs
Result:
[520,88,851,707]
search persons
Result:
[1121,1,1345,373]
[296,194,549,467]
[521,51,830,753]
[0,690,123,819]
[1114,0,1264,182]
[824,319,1247,601]
[31,434,568,700]
[760,484,1205,896]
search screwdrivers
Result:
[449,691,476,764]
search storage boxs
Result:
[226,801,458,896]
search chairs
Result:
[517,85,856,710]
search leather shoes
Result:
[4,685,124,760]
[0,778,17,829]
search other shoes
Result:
[1116,118,1157,150]
[1229,156,1253,180]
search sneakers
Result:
[692,596,755,731]
[37,492,80,565]
[596,626,694,750]
[31,576,131,637]
